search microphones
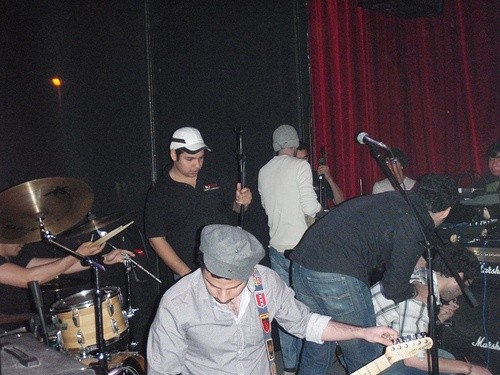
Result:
[356,132,387,150]
[317,158,324,180]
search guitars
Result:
[349,330,434,374]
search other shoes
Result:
[283,368,296,374]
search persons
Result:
[286,170,460,375]
[468,138,500,219]
[145,222,400,375]
[292,139,345,207]
[370,147,420,192]
[256,124,328,292]
[363,243,493,375]
[139,123,253,279]
[0,240,136,340]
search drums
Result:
[49,286,131,352]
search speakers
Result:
[441,279,500,375]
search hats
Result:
[199,225,265,280]
[169,127,211,153]
[272,124,299,151]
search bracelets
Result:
[463,355,474,375]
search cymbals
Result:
[0,177,95,245]
[51,211,130,243]
